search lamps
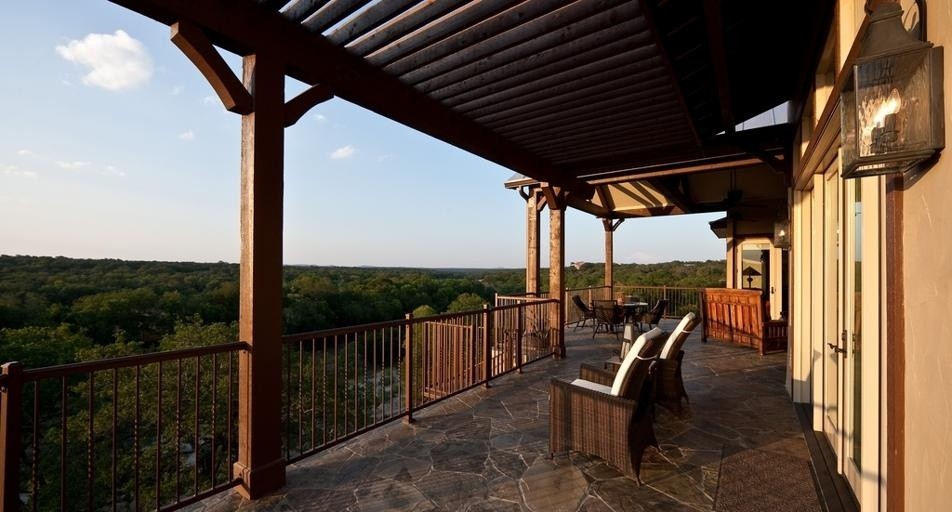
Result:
[841,45,943,180]
[743,266,763,287]
[774,222,792,248]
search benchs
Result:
[698,288,786,355]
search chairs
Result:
[605,312,703,409]
[571,293,669,340]
[551,326,665,487]
